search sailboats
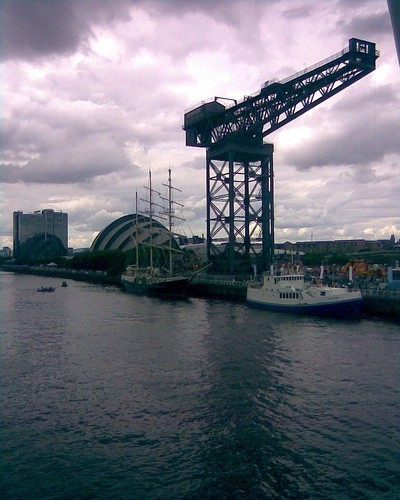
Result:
[120,165,191,295]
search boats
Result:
[245,266,363,317]
